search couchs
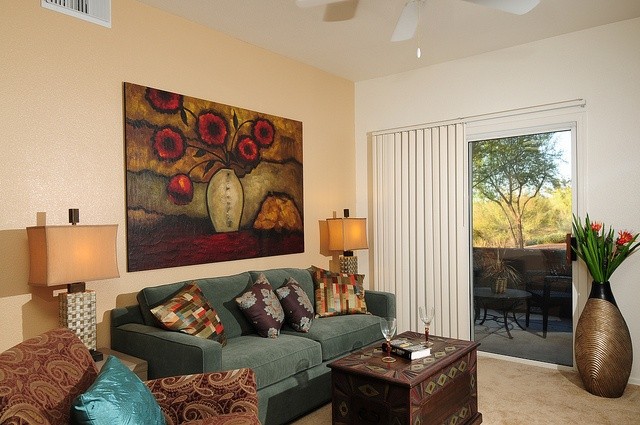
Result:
[111,267,397,425]
[1,327,259,425]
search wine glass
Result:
[418,307,435,347]
[379,317,397,363]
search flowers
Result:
[145,87,275,206]
[570,214,640,280]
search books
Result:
[382,337,432,361]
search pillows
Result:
[275,276,313,332]
[148,280,227,350]
[233,273,286,339]
[310,264,368,319]
[76,351,166,425]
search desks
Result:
[473,287,531,339]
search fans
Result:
[297,0,539,60]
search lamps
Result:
[27,207,122,360]
[326,208,370,274]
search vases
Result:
[206,168,245,233]
[491,278,508,294]
[574,280,633,397]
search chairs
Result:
[525,269,572,337]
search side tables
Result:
[93,343,149,383]
